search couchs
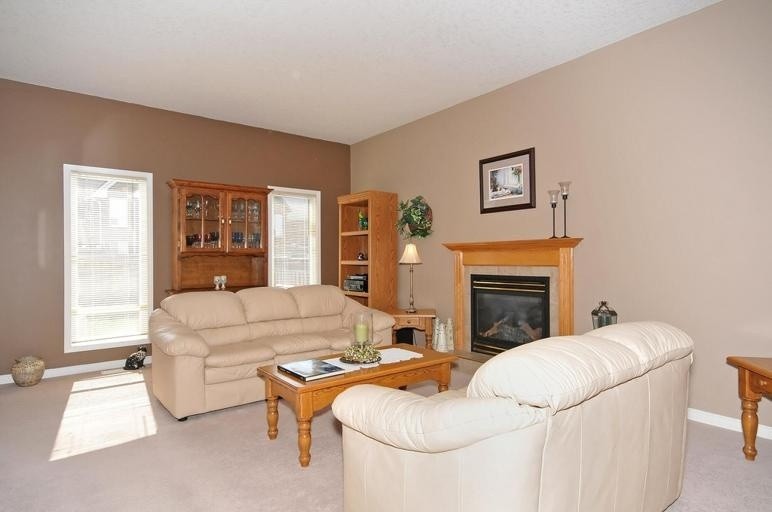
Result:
[331,320,695,512]
[147,284,396,422]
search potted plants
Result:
[392,195,434,240]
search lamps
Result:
[557,180,572,238]
[398,244,423,313]
[547,189,560,239]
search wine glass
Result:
[205,231,219,248]
[185,233,201,249]
[213,275,227,290]
[231,232,243,249]
[185,199,201,217]
[351,311,373,349]
[344,344,378,362]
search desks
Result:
[391,309,436,349]
[726,356,772,461]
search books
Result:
[277,359,346,382]
[343,275,368,292]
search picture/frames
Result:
[479,147,536,214]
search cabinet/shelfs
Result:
[166,176,274,297]
[337,190,398,316]
